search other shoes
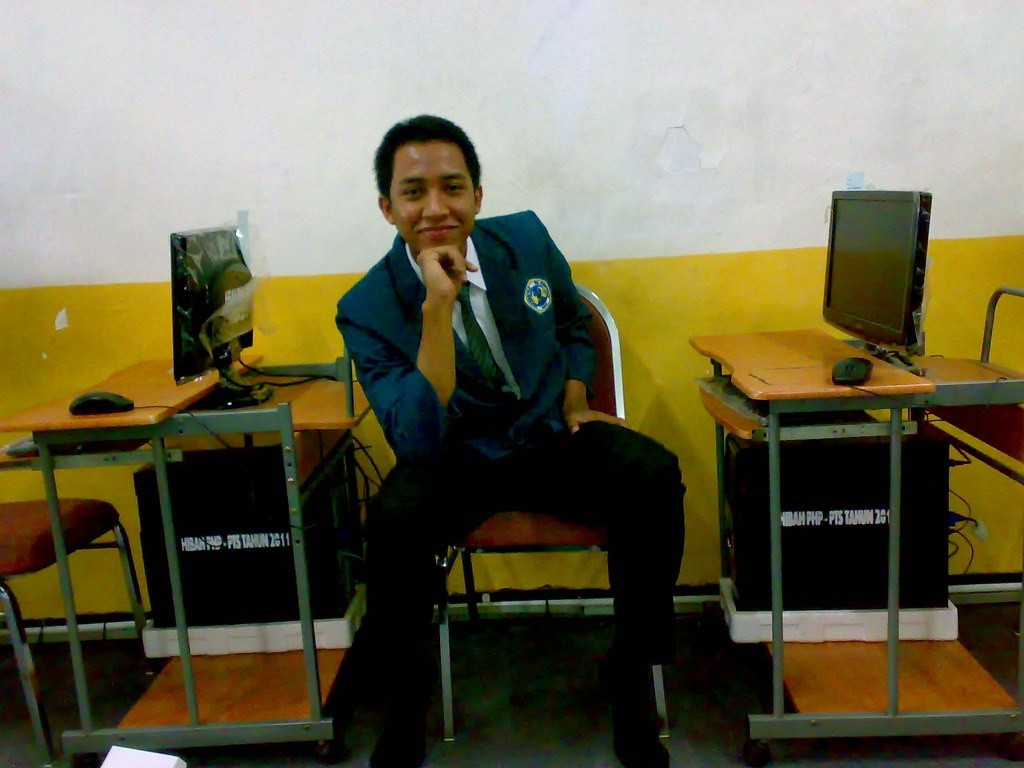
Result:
[612,690,671,768]
[368,727,426,768]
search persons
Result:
[334,115,687,768]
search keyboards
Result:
[0,435,40,458]
[695,375,770,426]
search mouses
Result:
[69,391,135,416]
[833,357,873,386]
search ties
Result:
[455,281,508,391]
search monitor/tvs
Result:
[823,188,932,363]
[170,224,271,408]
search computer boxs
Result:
[720,421,948,611]
[135,446,356,628]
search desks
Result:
[688,289,1024,768]
[0,356,373,768]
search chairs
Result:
[435,282,670,740]
[0,498,153,768]
